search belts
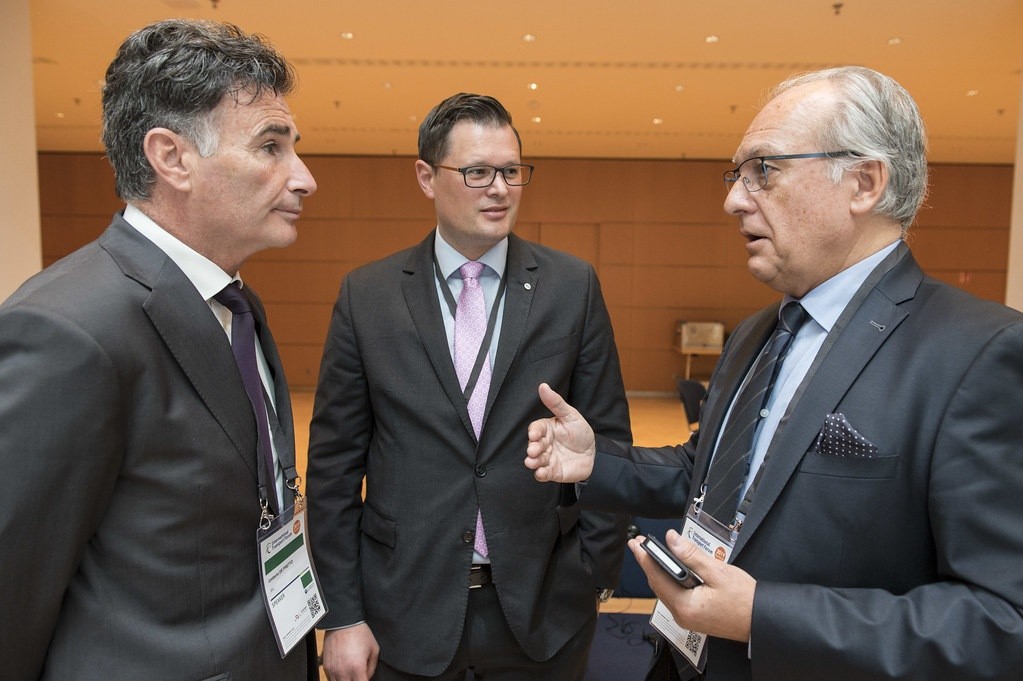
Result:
[468,564,492,588]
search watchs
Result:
[596,588,614,602]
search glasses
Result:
[428,163,534,188]
[723,150,862,194]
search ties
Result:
[453,260,493,559]
[213,282,280,516]
[667,302,807,681]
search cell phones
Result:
[645,541,685,578]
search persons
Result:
[0,19,319,681]
[525,65,1023,681]
[306,92,633,681]
